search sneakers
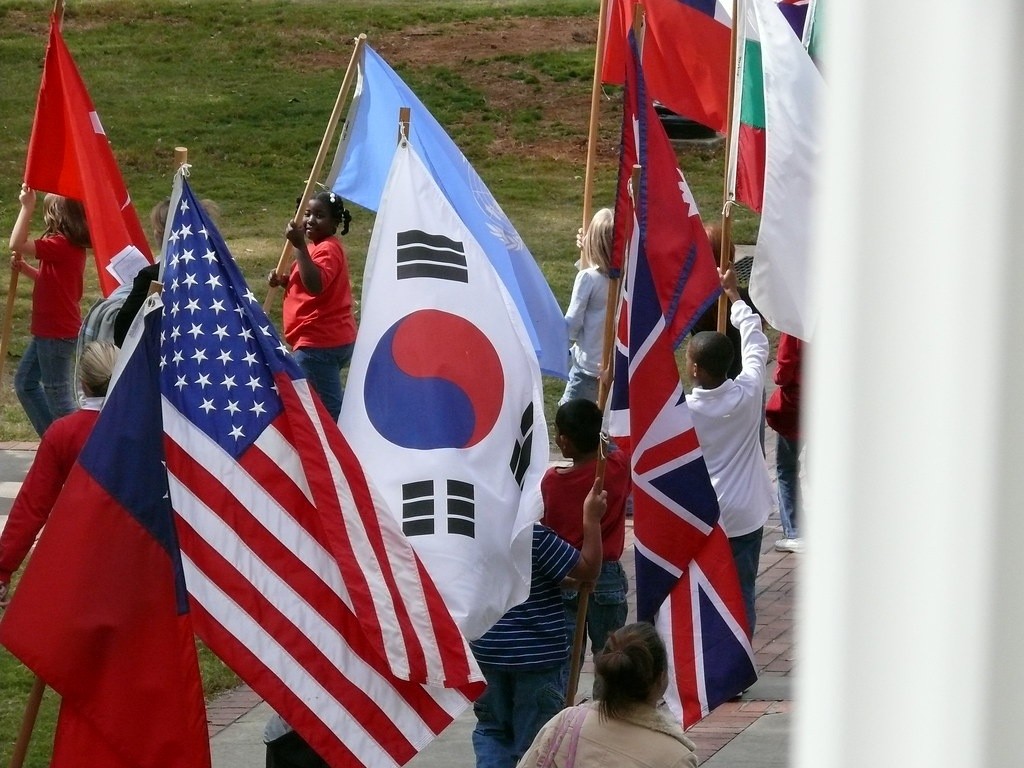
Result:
[775,539,802,554]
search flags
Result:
[572,0,832,735]
[158,168,488,768]
[336,136,550,641]
[0,291,213,768]
[24,15,154,299]
[322,44,570,382]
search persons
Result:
[516,621,698,768]
[557,207,615,425]
[9,183,93,439]
[113,198,219,349]
[539,399,633,693]
[268,191,358,424]
[469,477,608,768]
[765,330,806,553]
[689,222,766,461]
[0,340,121,609]
[685,260,769,698]
